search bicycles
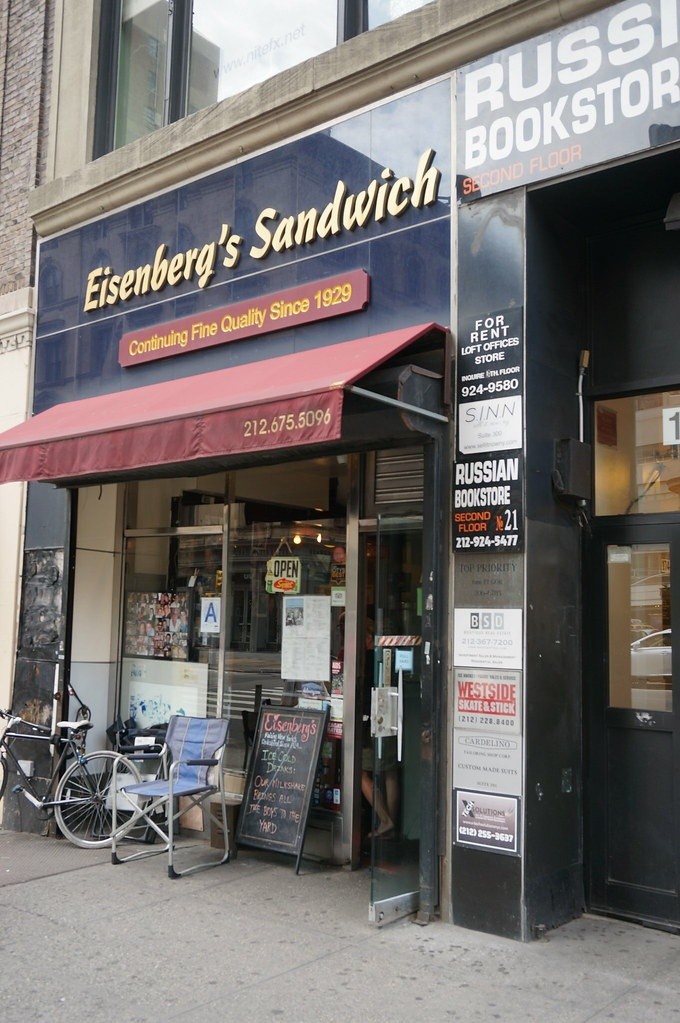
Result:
[0,710,146,850]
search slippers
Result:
[368,831,393,840]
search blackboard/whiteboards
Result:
[233,704,332,855]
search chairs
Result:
[111,715,230,879]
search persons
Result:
[336,613,401,842]
[124,592,187,658]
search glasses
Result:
[336,624,343,630]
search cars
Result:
[629,573,670,607]
[629,630,673,677]
[630,617,658,643]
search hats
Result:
[368,618,398,635]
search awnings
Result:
[0,322,450,483]
